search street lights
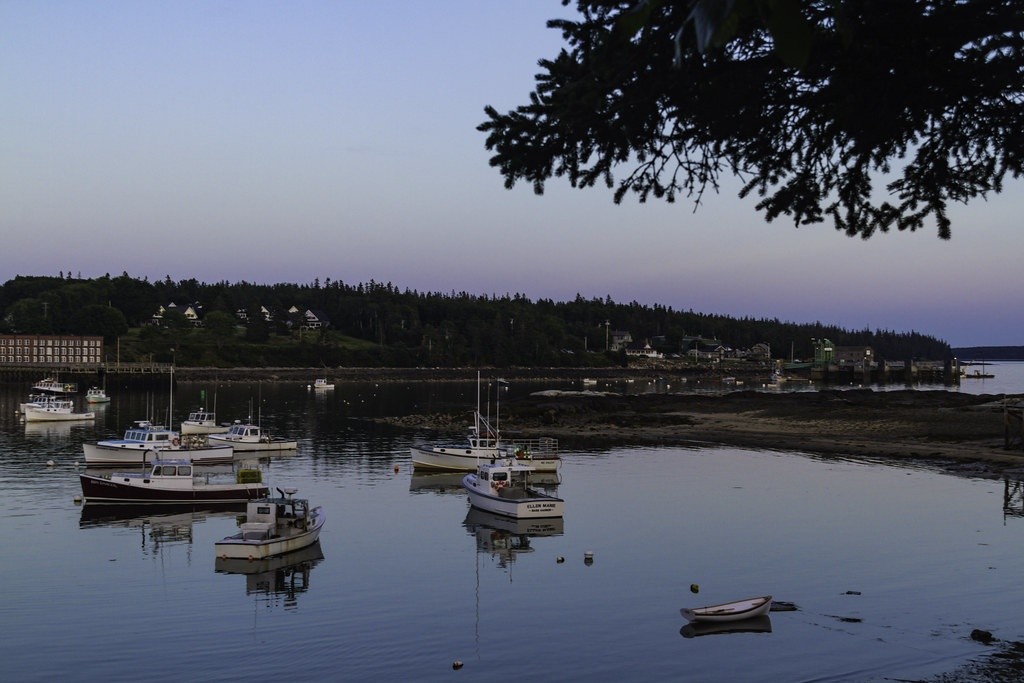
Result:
[605,320,610,352]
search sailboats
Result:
[461,369,567,518]
[458,505,565,582]
[408,375,564,471]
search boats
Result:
[309,376,336,389]
[214,487,326,556]
[679,614,773,639]
[23,420,97,443]
[18,392,96,421]
[213,537,325,615]
[30,369,78,396]
[75,495,270,573]
[680,593,775,626]
[82,366,236,467]
[73,407,276,505]
[86,386,111,403]
[207,380,298,451]
[406,467,563,493]
[178,374,234,435]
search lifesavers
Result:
[173,438,180,446]
[495,481,505,493]
